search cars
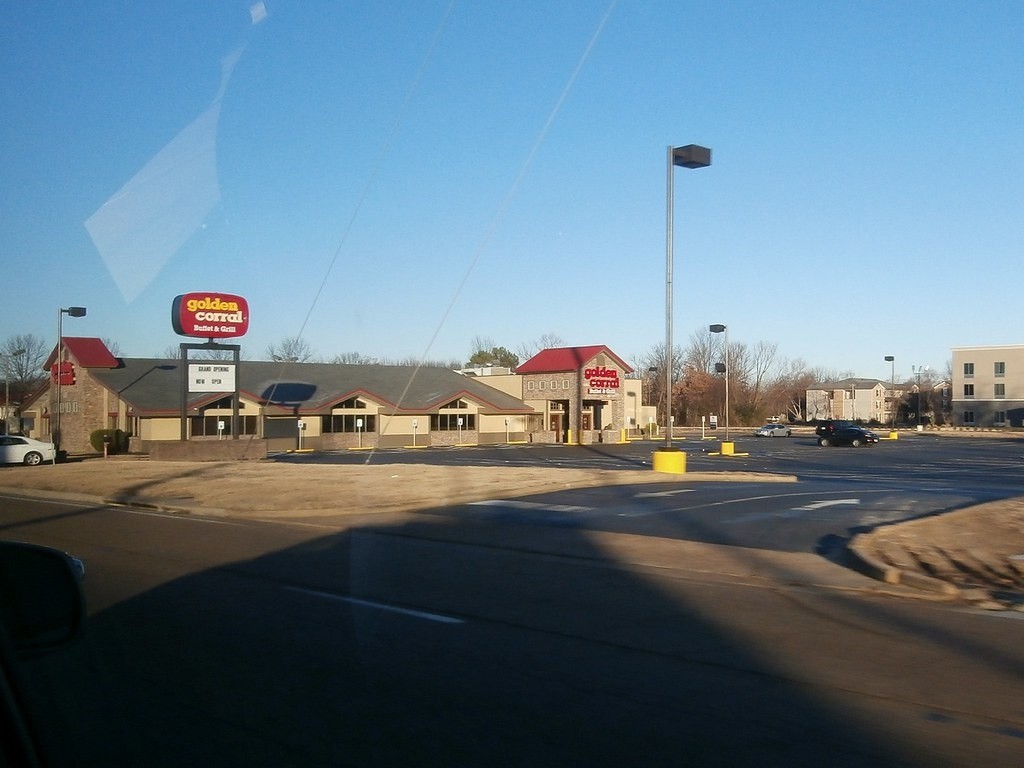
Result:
[0,434,56,466]
[754,424,791,437]
[818,428,879,447]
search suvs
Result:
[816,419,856,437]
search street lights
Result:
[663,144,714,451]
[5,349,27,435]
[709,324,728,442]
[647,366,657,405]
[54,306,87,452]
[885,355,895,431]
